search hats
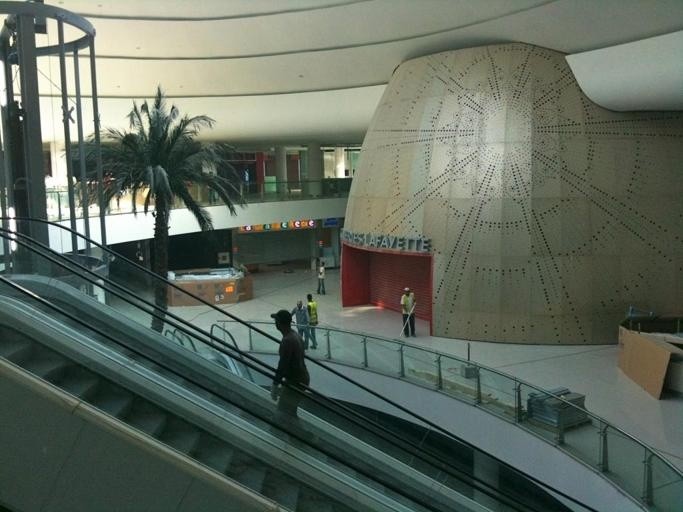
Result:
[271,310,292,323]
[403,288,410,292]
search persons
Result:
[290,300,308,351]
[207,171,216,205]
[400,287,417,338]
[315,261,326,295]
[270,310,311,417]
[305,294,319,349]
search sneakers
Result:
[310,346,316,349]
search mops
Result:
[390,301,419,345]
